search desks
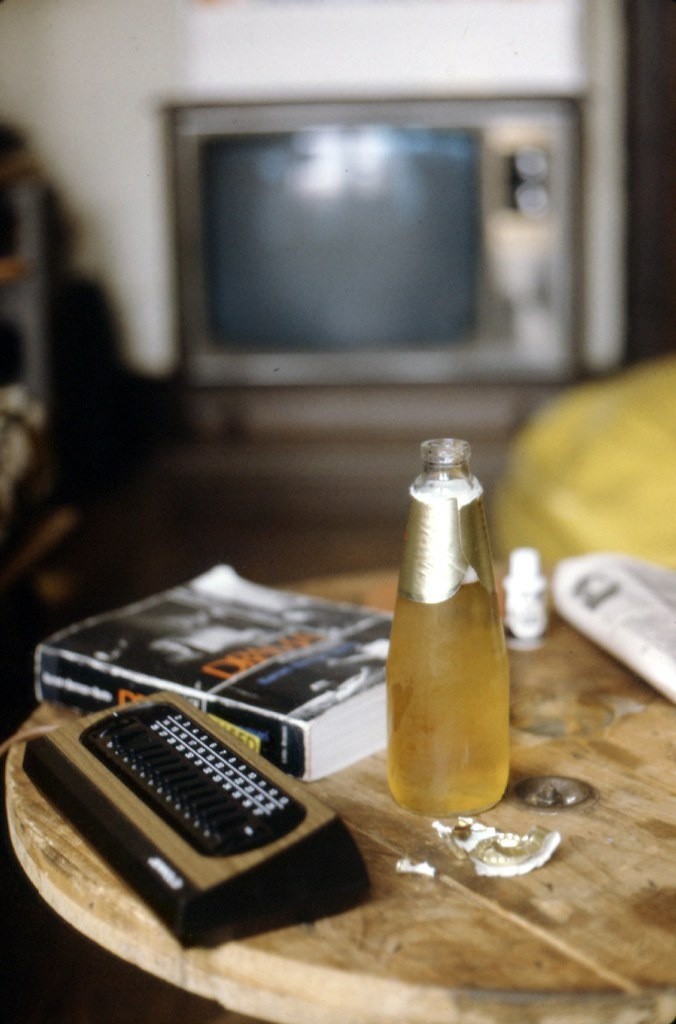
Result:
[4,567,676,1024]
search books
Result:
[35,565,393,780]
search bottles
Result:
[384,437,510,817]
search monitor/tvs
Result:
[167,96,586,388]
